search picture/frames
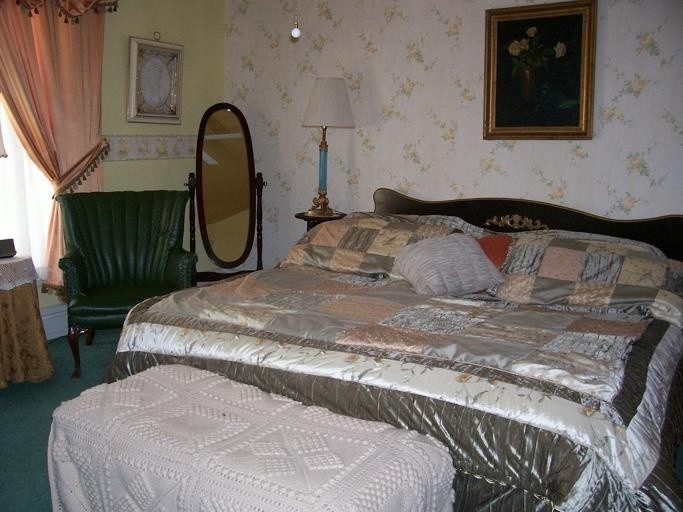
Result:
[124,36,184,126]
[481,1,597,142]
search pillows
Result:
[392,233,510,298]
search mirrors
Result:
[182,101,267,287]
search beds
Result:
[121,188,681,512]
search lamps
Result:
[300,76,356,216]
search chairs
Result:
[52,188,198,381]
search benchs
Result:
[49,363,455,512]
